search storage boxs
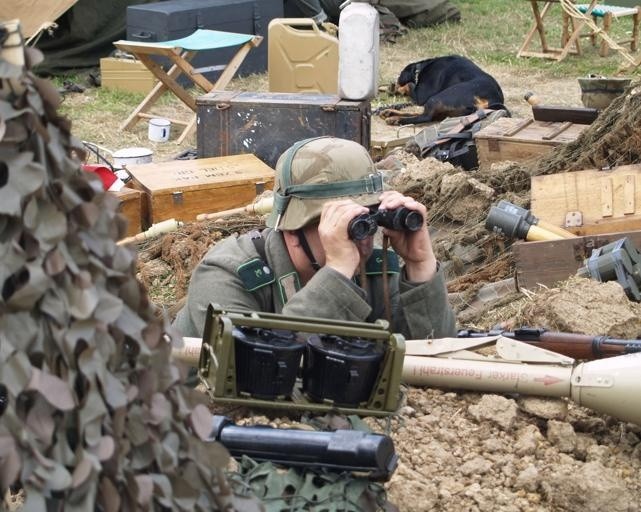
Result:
[196,91,370,171]
[512,165,641,292]
[112,187,141,237]
[473,117,590,168]
[124,153,276,226]
[126,1,284,87]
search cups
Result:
[147,118,171,142]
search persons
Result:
[171,134,459,381]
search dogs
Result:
[377,52,512,125]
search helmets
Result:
[265,135,383,230]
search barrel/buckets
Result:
[267,17,339,94]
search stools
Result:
[561,4,641,57]
[516,0,597,62]
[113,29,264,145]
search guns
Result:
[458,326,641,357]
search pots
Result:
[82,139,154,167]
